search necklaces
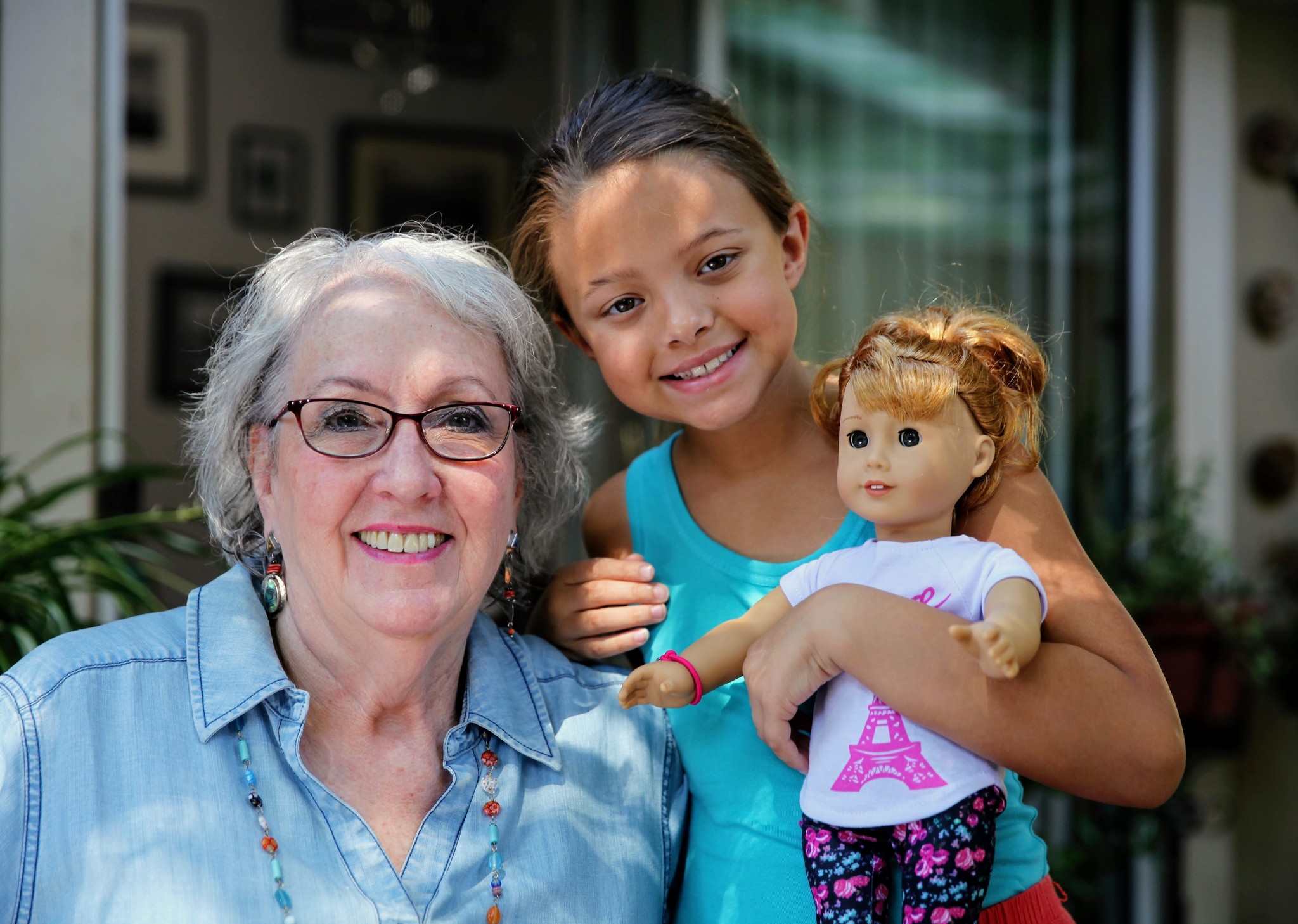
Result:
[227,718,505,924]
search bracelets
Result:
[658,651,702,706]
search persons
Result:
[616,303,1049,924]
[0,227,692,924]
[518,66,1185,924]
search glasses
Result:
[266,398,522,461]
